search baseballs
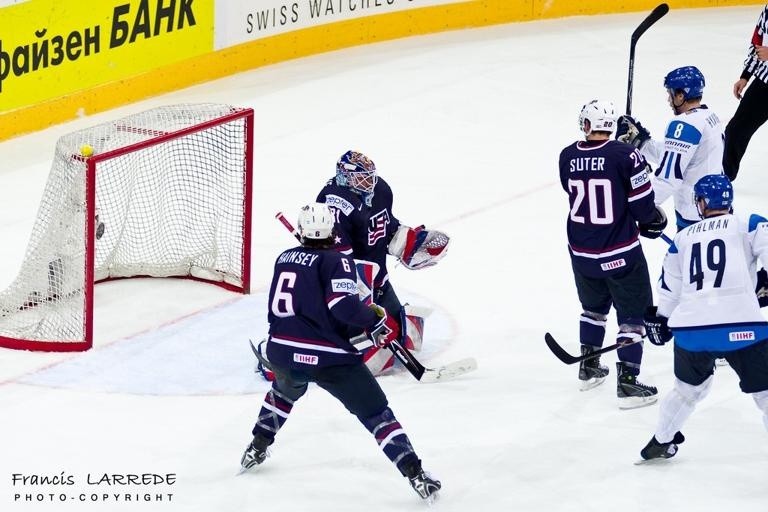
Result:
[82,144,94,156]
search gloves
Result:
[635,205,667,239]
[641,306,674,347]
[615,113,652,151]
[366,303,398,349]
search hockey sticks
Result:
[625,3,668,116]
[275,211,476,383]
[544,333,647,364]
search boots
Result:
[616,362,656,398]
[640,431,685,460]
[401,460,441,499]
[577,343,609,381]
[241,431,271,468]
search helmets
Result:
[296,202,334,240]
[335,150,379,196]
[578,99,619,137]
[693,174,734,209]
[663,66,705,100]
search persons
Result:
[615,66,726,232]
[640,174,768,460]
[240,202,441,499]
[258,151,449,382]
[722,3,768,181]
[558,99,667,398]
